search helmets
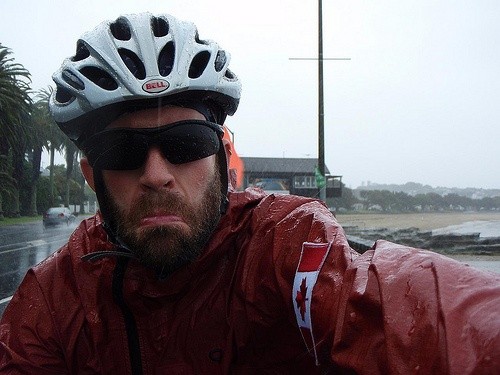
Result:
[47,12,243,141]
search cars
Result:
[44,206,76,227]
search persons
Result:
[1,16,500,375]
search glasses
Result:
[78,121,226,170]
